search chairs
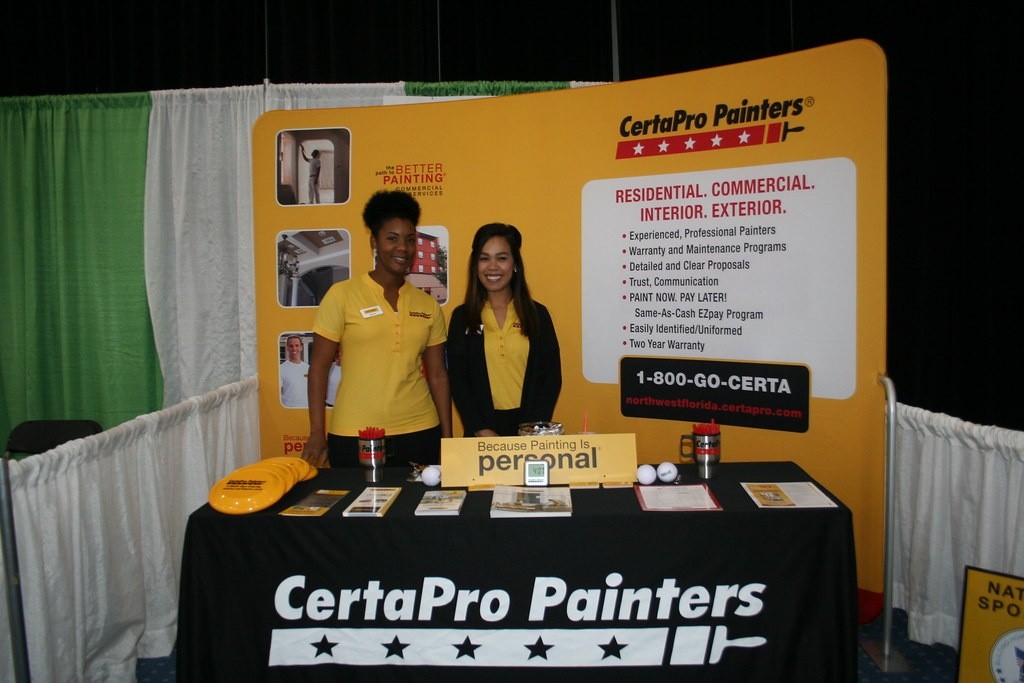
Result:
[3,419,104,457]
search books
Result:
[489,485,572,518]
[414,490,465,515]
[342,487,402,517]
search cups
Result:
[518,422,566,436]
[681,432,721,479]
[358,438,395,482]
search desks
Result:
[172,460,859,683]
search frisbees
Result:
[208,456,318,514]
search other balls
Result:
[421,465,443,486]
[637,462,656,486]
[657,460,679,483]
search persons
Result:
[300,143,321,204]
[279,336,343,408]
[297,191,453,468]
[446,223,562,437]
